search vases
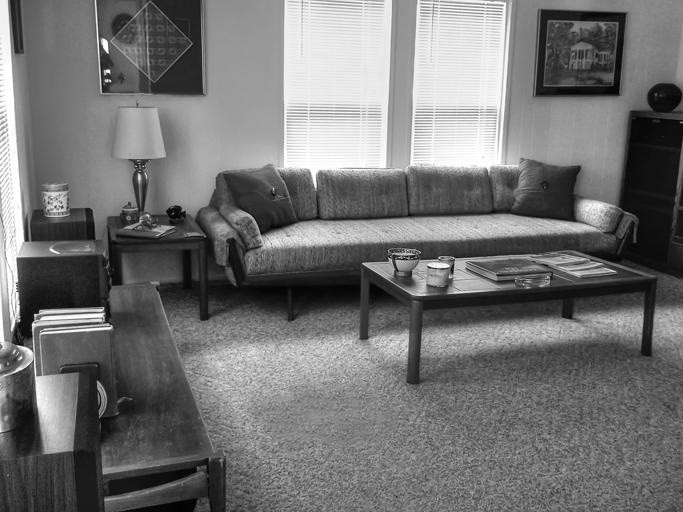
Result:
[645,82,682,114]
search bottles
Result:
[119,201,137,226]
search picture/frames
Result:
[93,1,206,98]
[8,0,26,55]
[533,8,628,97]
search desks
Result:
[104,212,210,322]
[15,281,215,511]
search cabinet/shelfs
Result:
[613,110,683,281]
[0,368,106,511]
[28,206,96,241]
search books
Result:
[32,307,119,418]
[555,262,604,271]
[528,252,590,266]
[549,267,618,279]
[465,259,553,282]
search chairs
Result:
[103,449,228,511]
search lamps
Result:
[108,99,167,225]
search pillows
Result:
[510,157,584,223]
[217,162,298,236]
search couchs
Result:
[194,157,642,322]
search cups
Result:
[41,184,70,219]
[426,262,449,287]
[387,248,420,277]
[437,255,456,279]
